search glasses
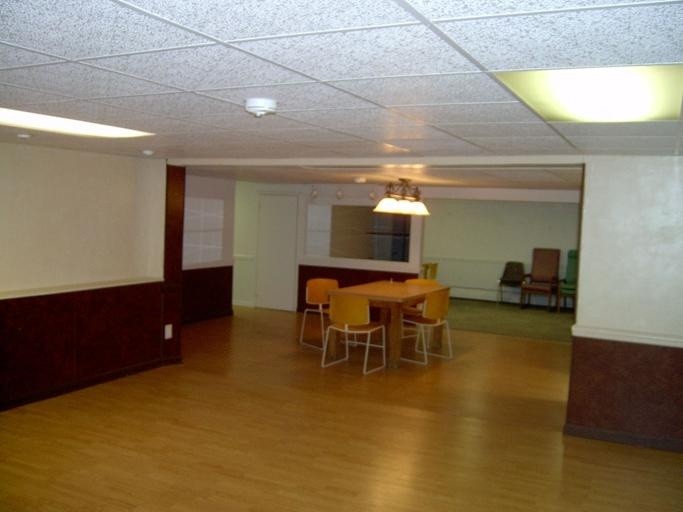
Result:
[246,98,278,118]
[373,177,431,215]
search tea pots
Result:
[296,198,421,274]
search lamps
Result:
[521,248,560,311]
[556,249,577,314]
[299,277,453,375]
[497,261,527,306]
